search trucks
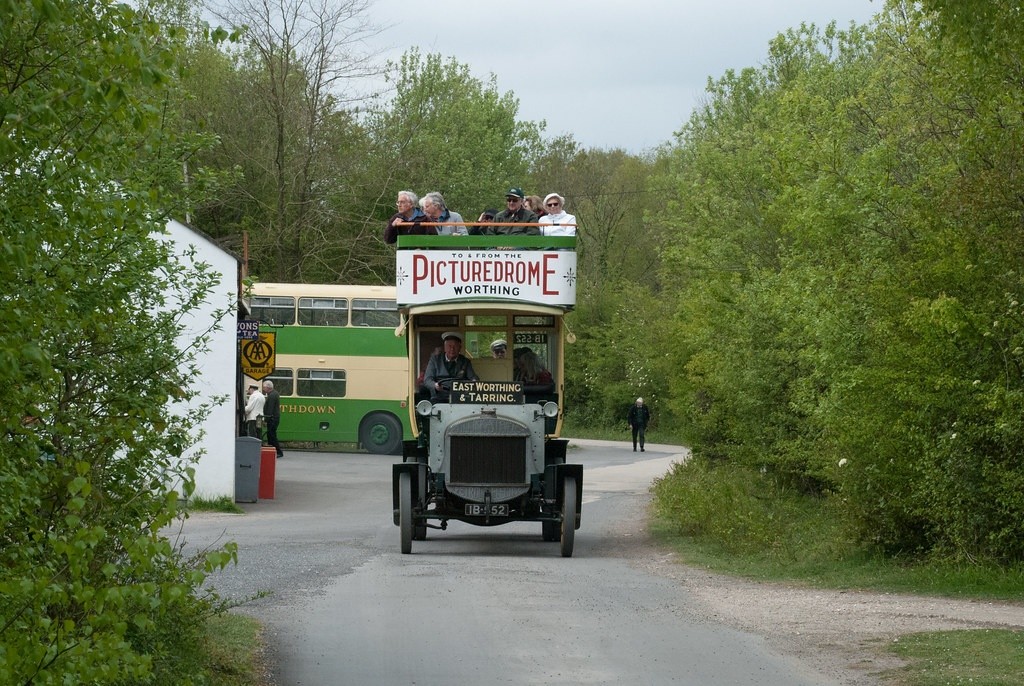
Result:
[392,222,582,558]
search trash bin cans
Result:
[235,435,263,502]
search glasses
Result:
[507,199,518,202]
[548,203,558,207]
[484,216,493,220]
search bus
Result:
[240,282,481,455]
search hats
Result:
[442,332,462,342]
[505,187,524,198]
[484,209,498,216]
[490,340,507,351]
[543,193,564,212]
[249,384,258,389]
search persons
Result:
[423,332,553,406]
[245,380,284,458]
[628,397,650,452]
[384,187,576,244]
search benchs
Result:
[469,358,513,382]
[418,383,558,435]
